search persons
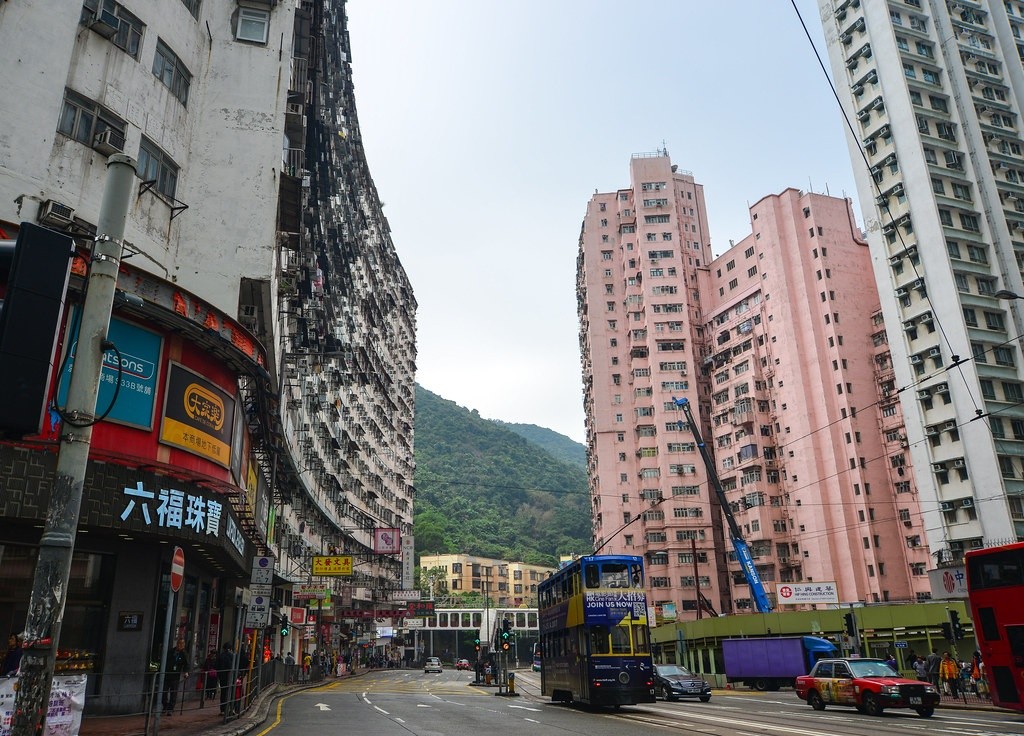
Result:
[162,639,406,716]
[885,647,992,701]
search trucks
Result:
[722,634,844,693]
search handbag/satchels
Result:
[977,680,989,694]
[970,677,977,684]
[944,681,952,696]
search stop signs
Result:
[171,547,185,593]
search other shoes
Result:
[167,712,172,716]
[162,709,166,715]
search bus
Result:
[963,540,1024,714]
[536,512,656,711]
[532,642,541,673]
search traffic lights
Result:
[949,608,966,640]
[475,638,480,651]
[503,620,511,632]
[941,621,955,646]
[502,632,510,651]
[843,613,854,637]
[281,616,288,636]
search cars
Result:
[456,659,470,670]
[424,656,443,673]
[794,657,941,720]
[652,662,712,703]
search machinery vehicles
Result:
[671,395,774,614]
